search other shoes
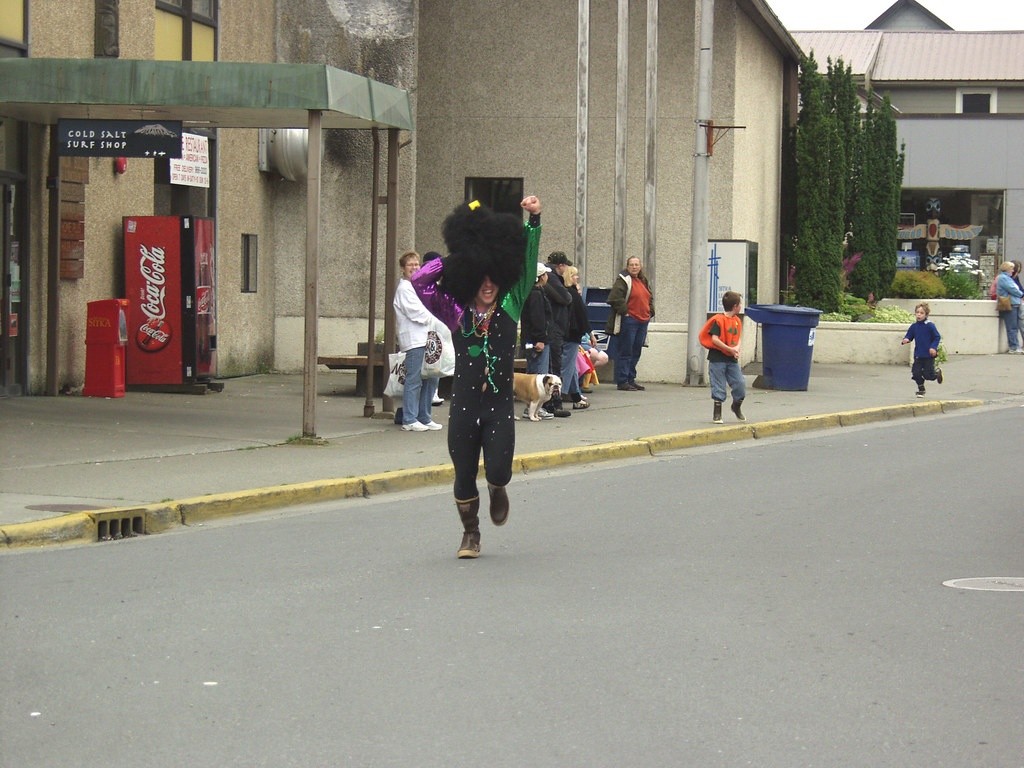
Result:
[936,368,942,384]
[547,409,571,416]
[573,400,589,409]
[1008,348,1024,355]
[916,385,926,397]
[617,380,637,391]
[431,388,443,405]
[629,381,645,389]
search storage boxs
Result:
[978,237,1003,297]
[895,249,921,271]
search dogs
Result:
[513,373,563,422]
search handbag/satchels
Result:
[995,296,1012,312]
[382,351,406,398]
[420,321,455,378]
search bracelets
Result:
[588,332,594,337]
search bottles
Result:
[194,254,214,362]
[142,321,164,346]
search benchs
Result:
[317,355,529,397]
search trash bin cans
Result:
[744,303,823,390]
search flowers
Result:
[935,253,985,299]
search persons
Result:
[994,260,1024,354]
[699,291,747,424]
[901,303,943,398]
[393,249,444,431]
[412,195,541,559]
[606,255,655,390]
[522,250,609,419]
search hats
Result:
[537,262,552,276]
[548,250,573,266]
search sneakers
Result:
[425,421,443,431]
[401,421,429,431]
[523,407,554,419]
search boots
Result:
[713,401,723,423]
[731,397,746,422]
[454,492,481,558]
[487,470,511,526]
